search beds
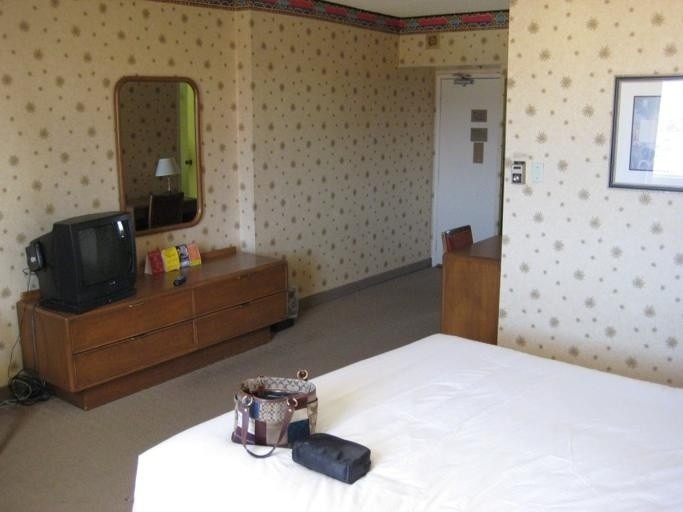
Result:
[133,332,683,512]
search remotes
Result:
[173,274,186,286]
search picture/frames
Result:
[608,75,683,192]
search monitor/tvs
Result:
[30,211,137,316]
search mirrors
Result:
[112,75,203,239]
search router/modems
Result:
[25,243,44,272]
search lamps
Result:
[155,156,179,195]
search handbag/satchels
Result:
[231,368,318,458]
[292,433,370,484]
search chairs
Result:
[148,193,184,228]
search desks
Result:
[126,196,195,231]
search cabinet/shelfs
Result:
[15,246,288,410]
